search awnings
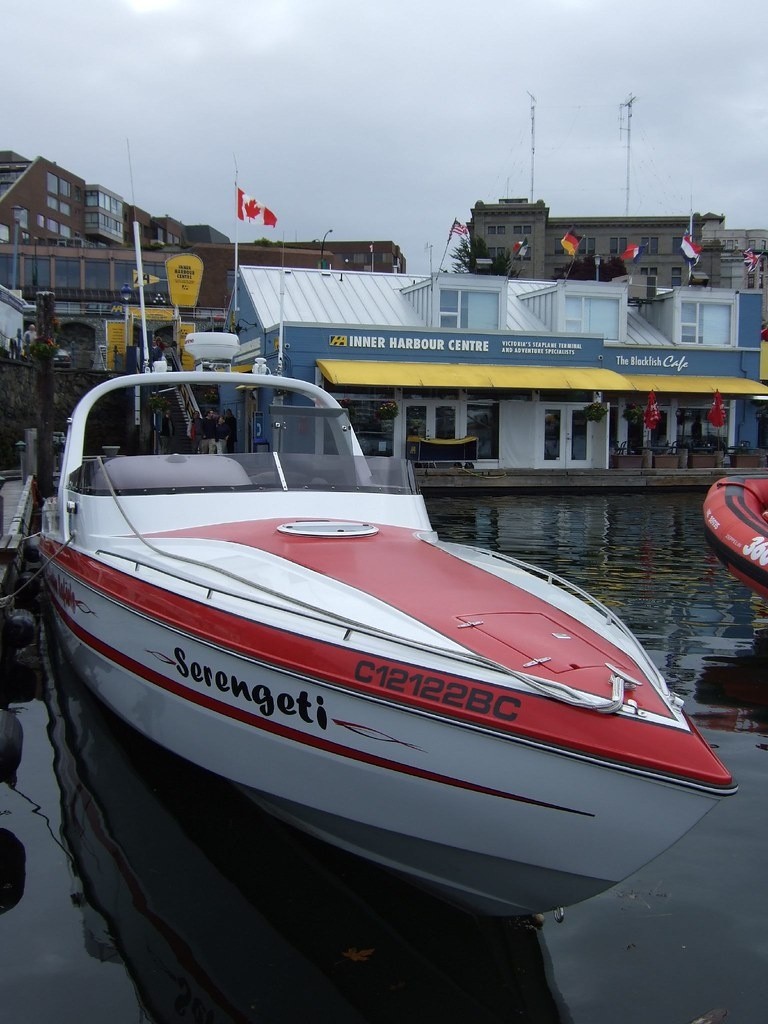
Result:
[314,358,635,390]
[622,375,768,393]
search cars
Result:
[53,348,72,368]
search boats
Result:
[26,134,742,923]
[36,612,572,1024]
[705,473,768,605]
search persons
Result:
[23,324,37,343]
[154,343,165,361]
[160,410,176,454]
[187,409,238,454]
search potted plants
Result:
[653,449,681,469]
[690,450,717,468]
[611,452,644,469]
[728,447,761,468]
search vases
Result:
[380,420,393,433]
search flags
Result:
[621,242,648,263]
[515,236,528,256]
[561,229,579,255]
[452,221,468,235]
[237,188,277,228]
[680,235,702,266]
[743,248,762,272]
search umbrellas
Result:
[644,389,662,450]
[707,389,726,453]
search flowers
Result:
[24,335,60,375]
[621,401,645,426]
[375,400,399,420]
[583,402,607,424]
[145,393,172,418]
[203,385,220,404]
